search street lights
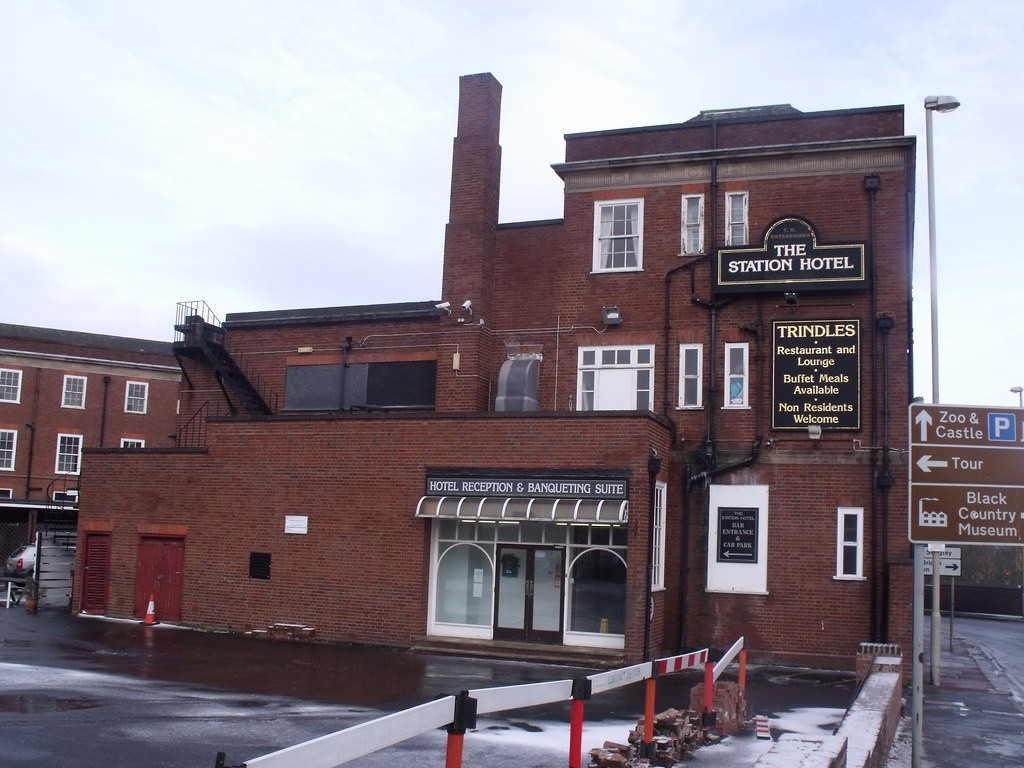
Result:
[921,95,962,688]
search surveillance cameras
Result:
[462,300,472,310]
[435,302,450,309]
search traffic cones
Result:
[139,593,160,626]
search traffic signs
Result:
[909,402,1024,546]
[921,546,961,577]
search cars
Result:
[7,544,36,575]
